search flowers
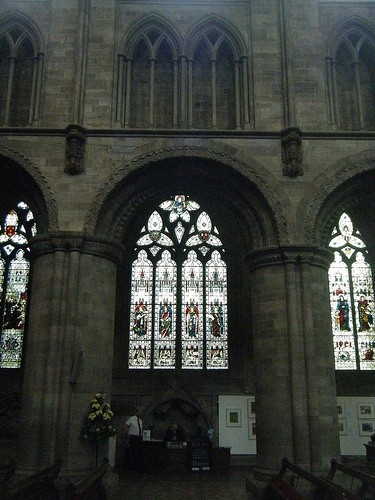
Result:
[78,387,118,442]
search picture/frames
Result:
[337,402,345,417]
[226,409,241,427]
[248,419,256,440]
[359,420,375,436]
[247,398,256,418]
[358,403,374,419]
[338,419,347,435]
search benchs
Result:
[269,456,353,500]
[0,458,109,500]
[315,457,375,500]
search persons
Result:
[164,420,186,443]
[123,408,146,472]
